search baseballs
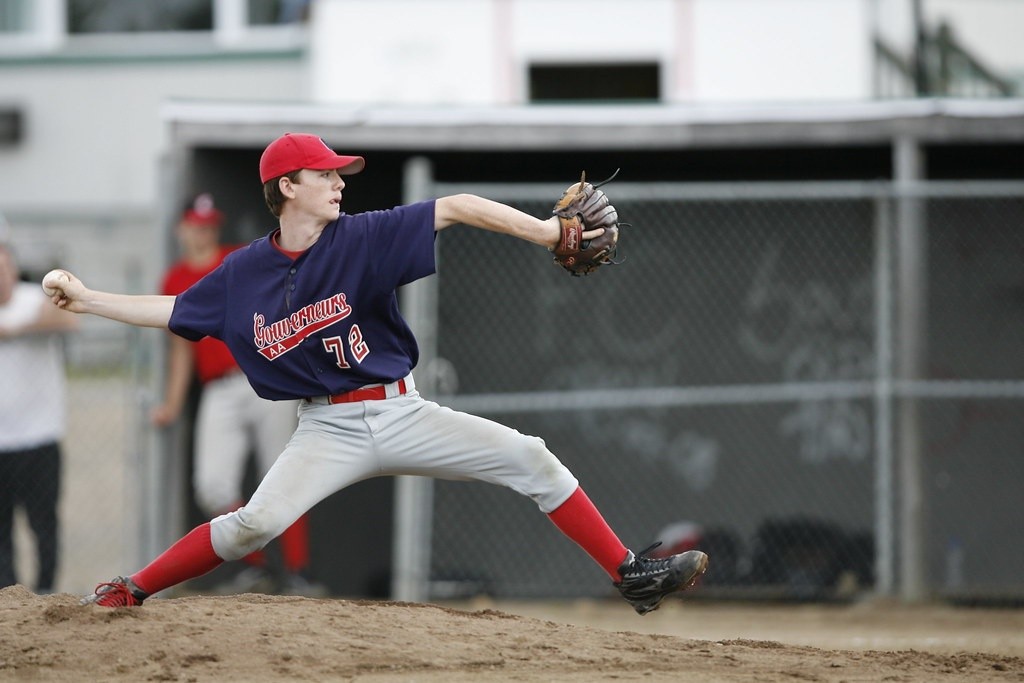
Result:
[42,267,72,296]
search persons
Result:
[42,131,710,617]
[0,190,314,598]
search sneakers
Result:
[610,541,708,616]
[76,574,149,610]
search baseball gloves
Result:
[554,165,620,273]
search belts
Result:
[331,380,407,405]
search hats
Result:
[185,195,226,227]
[260,133,366,185]
